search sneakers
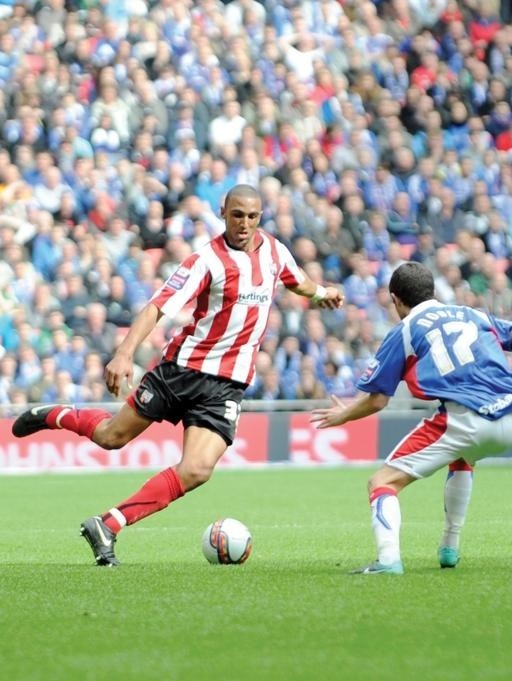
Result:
[78,513,122,567]
[347,558,406,576]
[11,402,77,438]
[437,542,462,568]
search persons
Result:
[308,261,512,575]
[12,184,346,567]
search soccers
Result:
[202,517,252,565]
